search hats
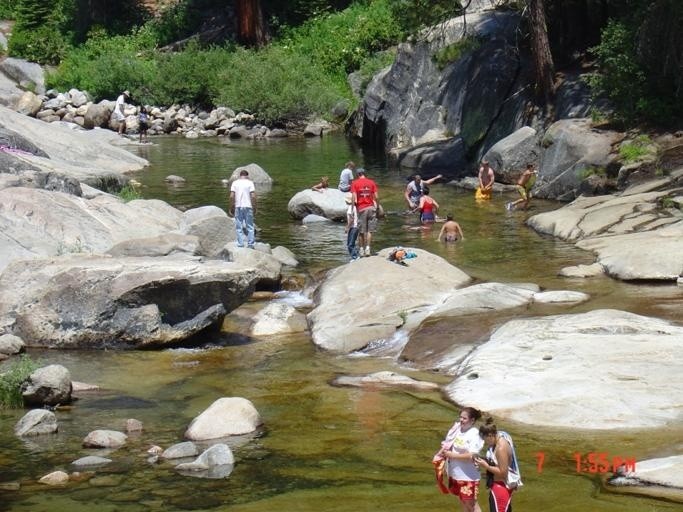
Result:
[344,192,352,203]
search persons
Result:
[472,416,523,512]
[227,170,258,250]
[310,176,329,194]
[430,406,485,511]
[113,90,130,137]
[506,163,538,211]
[516,163,537,211]
[473,158,495,199]
[137,106,148,143]
[350,168,380,258]
[342,193,360,263]
[411,186,439,224]
[336,161,356,192]
[435,212,464,243]
[404,173,443,214]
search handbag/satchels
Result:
[503,470,523,489]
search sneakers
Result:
[349,250,370,262]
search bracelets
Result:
[376,208,379,210]
